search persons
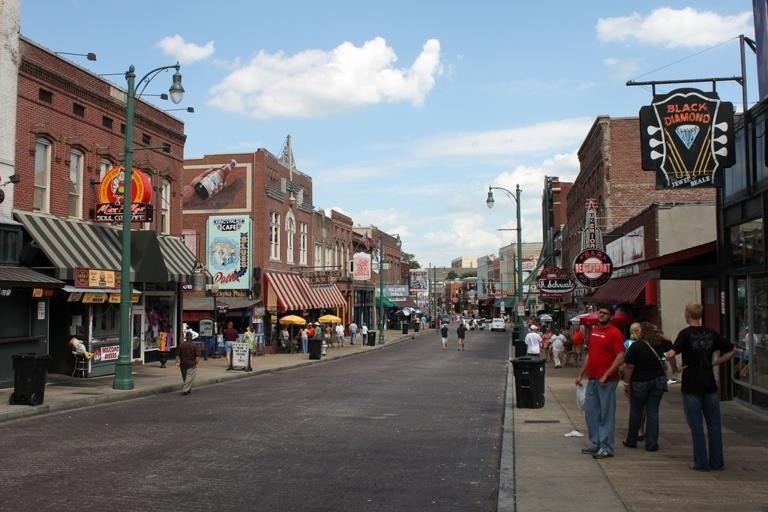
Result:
[176,332,200,395]
[622,321,673,452]
[242,326,255,368]
[575,303,626,459]
[666,302,737,471]
[619,322,646,441]
[524,314,586,368]
[277,313,469,355]
[223,321,240,370]
[183,168,226,205]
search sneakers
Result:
[181,390,192,395]
[580,446,614,459]
[624,433,659,452]
[688,462,726,472]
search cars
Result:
[437,311,506,332]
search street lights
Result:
[111,57,185,391]
[484,182,527,360]
[376,233,402,345]
[207,284,218,337]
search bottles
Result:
[195,159,237,200]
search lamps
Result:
[99,70,137,81]
[54,50,98,62]
[133,92,171,101]
[162,107,195,114]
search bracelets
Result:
[624,383,630,386]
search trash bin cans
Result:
[9,353,46,405]
[368,332,375,346]
[309,339,321,359]
[432,321,435,327]
[403,324,408,334]
[510,356,546,409]
[414,323,419,332]
[511,326,527,358]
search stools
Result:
[71,350,90,380]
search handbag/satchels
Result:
[658,357,673,382]
[575,382,588,411]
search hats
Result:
[184,332,193,340]
[307,321,321,326]
[530,325,539,330]
